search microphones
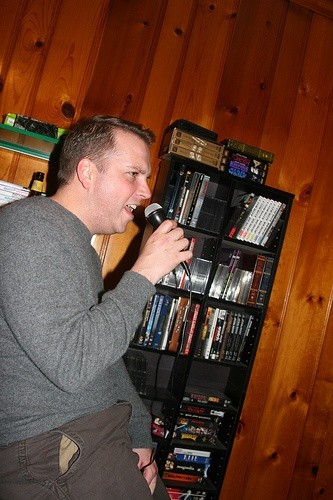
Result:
[144,203,191,276]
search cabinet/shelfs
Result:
[121,153,296,500]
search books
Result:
[3,112,65,139]
[162,385,231,500]
[0,179,46,207]
[132,127,287,362]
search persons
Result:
[0,114,194,500]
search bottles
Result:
[28,171,45,197]
